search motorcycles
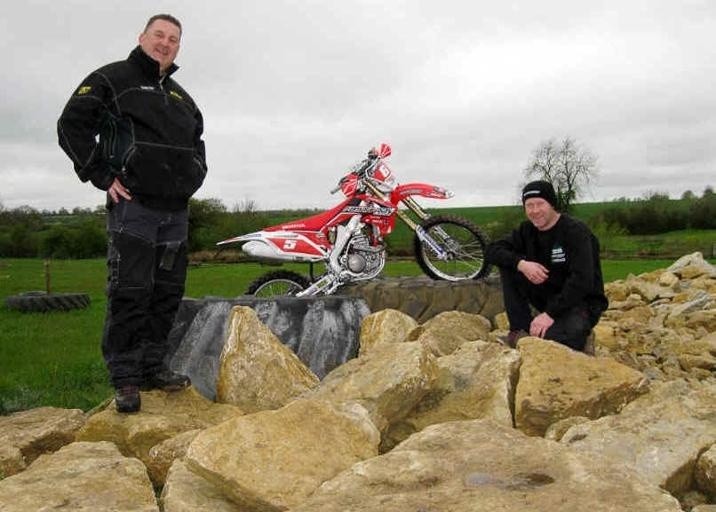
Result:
[212,141,492,298]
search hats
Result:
[522,180,557,209]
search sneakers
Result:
[115,369,191,413]
[584,331,595,354]
[495,330,528,348]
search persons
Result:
[484,181,608,352]
[57,14,208,413]
